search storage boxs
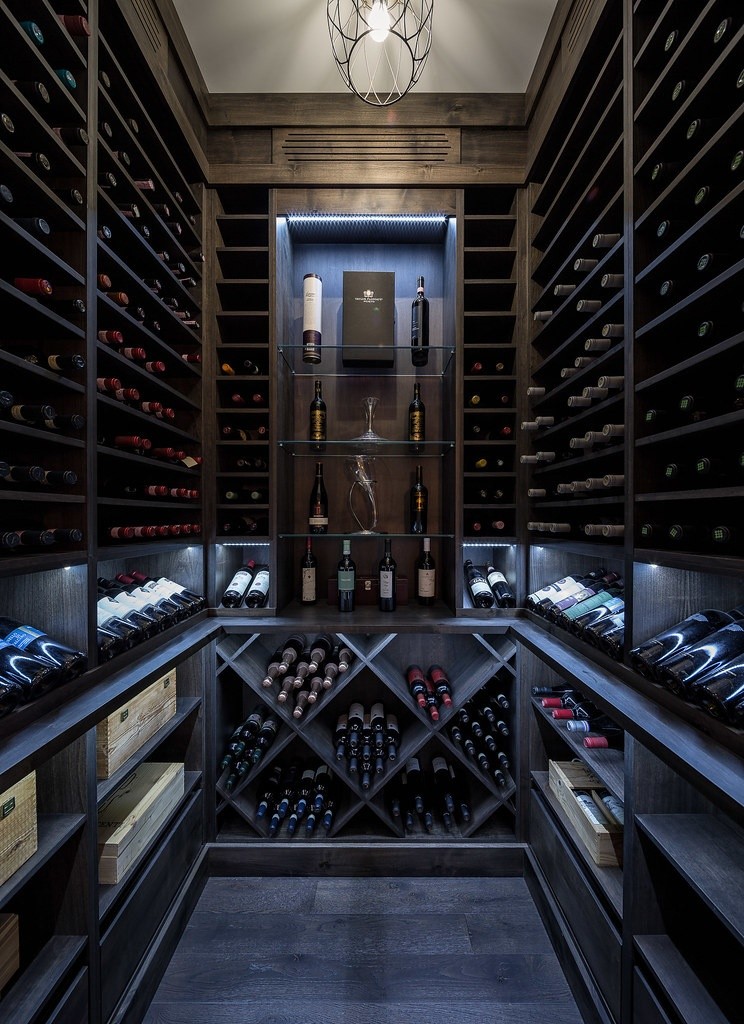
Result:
[96,762,186,884]
[0,770,37,888]
[96,667,176,777]
[548,756,624,867]
[0,913,20,992]
[342,271,396,368]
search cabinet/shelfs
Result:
[0,0,744,1024]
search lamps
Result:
[326,0,433,108]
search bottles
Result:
[0,16,205,501]
[219,424,268,442]
[0,612,90,720]
[526,567,626,665]
[99,521,203,547]
[486,560,517,608]
[519,228,627,547]
[221,359,265,377]
[299,536,318,606]
[417,537,436,610]
[309,379,327,454]
[377,539,397,612]
[336,539,355,612]
[530,678,625,760]
[95,569,208,665]
[0,523,83,558]
[219,486,266,537]
[409,464,429,535]
[219,632,511,835]
[465,361,514,537]
[631,15,744,556]
[628,600,744,733]
[220,559,256,609]
[245,563,270,609]
[465,558,495,609]
[408,382,425,454]
[302,273,322,364]
[574,786,625,825]
[236,456,267,472]
[229,392,266,408]
[309,461,329,536]
[409,275,429,366]
